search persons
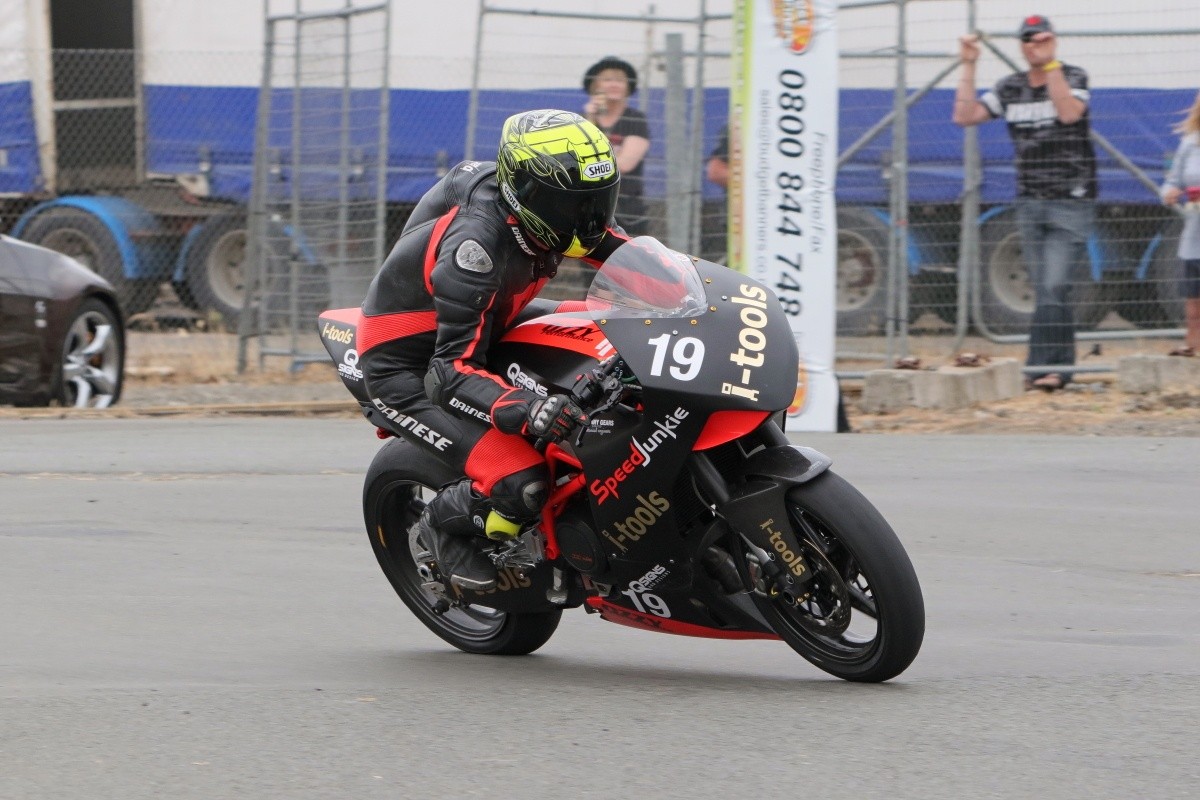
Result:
[705,137,729,188]
[579,55,654,238]
[951,14,1100,392]
[354,108,707,597]
[1159,91,1200,358]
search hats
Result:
[584,56,637,93]
[1017,16,1050,35]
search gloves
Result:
[528,393,591,445]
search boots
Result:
[420,479,499,591]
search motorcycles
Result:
[317,235,926,683]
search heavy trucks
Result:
[0,79,1200,337]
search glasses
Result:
[1021,36,1030,42]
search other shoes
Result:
[1034,369,1072,386]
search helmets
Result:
[496,109,621,258]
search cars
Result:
[0,233,126,408]
